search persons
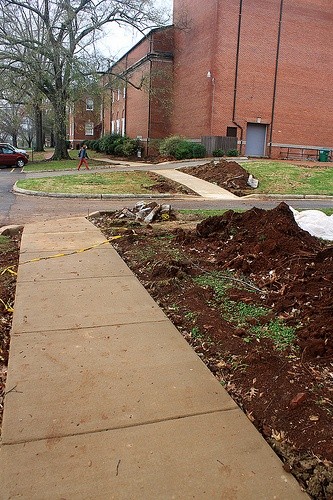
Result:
[77,145,91,171]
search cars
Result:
[0,142,28,155]
[0,145,28,167]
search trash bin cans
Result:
[317,148,330,162]
